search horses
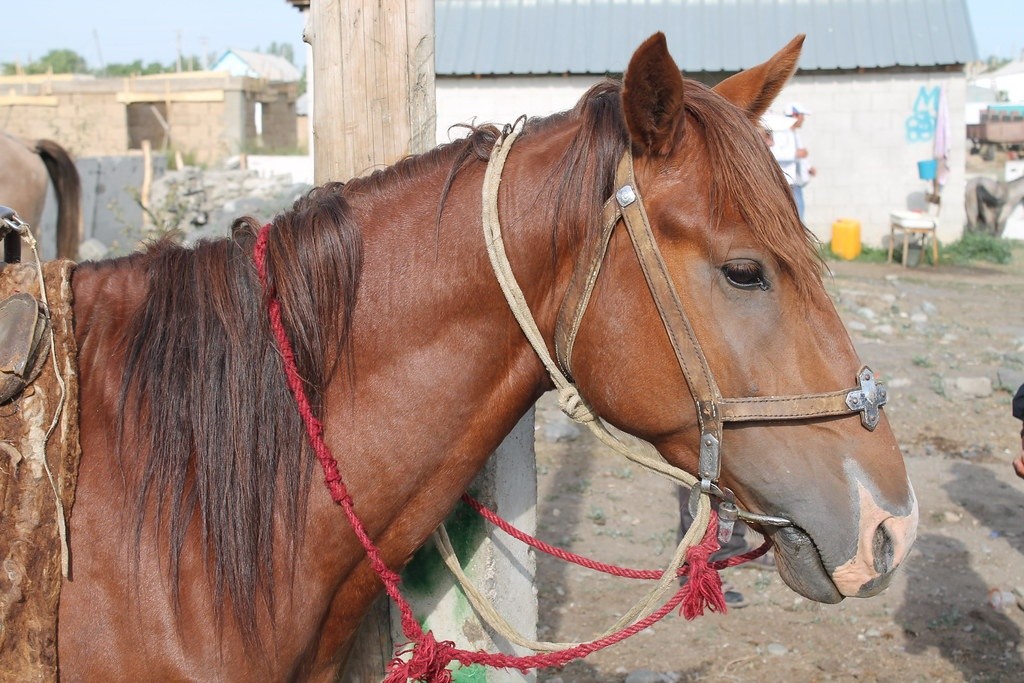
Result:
[0,32,919,683]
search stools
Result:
[888,224,939,271]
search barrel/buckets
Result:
[831,219,861,259]
